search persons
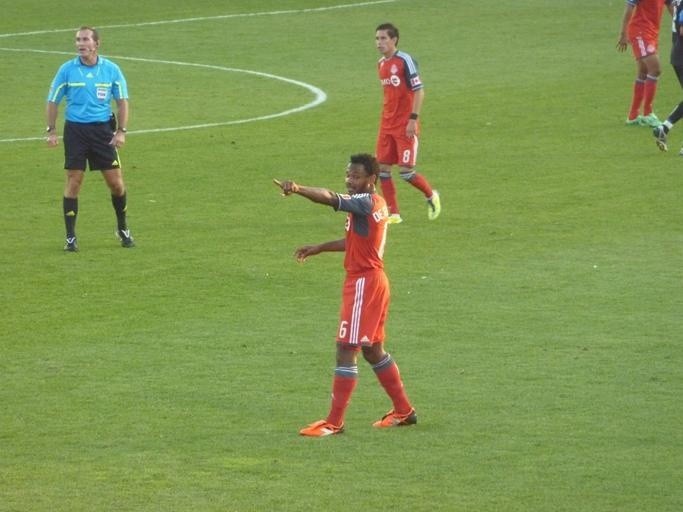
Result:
[45,26,136,251]
[271,153,416,435]
[653,2,682,152]
[617,1,665,127]
[372,23,443,225]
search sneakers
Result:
[426,189,440,219]
[115,230,134,247]
[373,408,416,426]
[300,420,344,436]
[388,214,403,224]
[625,113,666,150]
[64,236,77,252]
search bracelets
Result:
[408,112,418,120]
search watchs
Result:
[117,127,126,134]
[46,125,54,133]
